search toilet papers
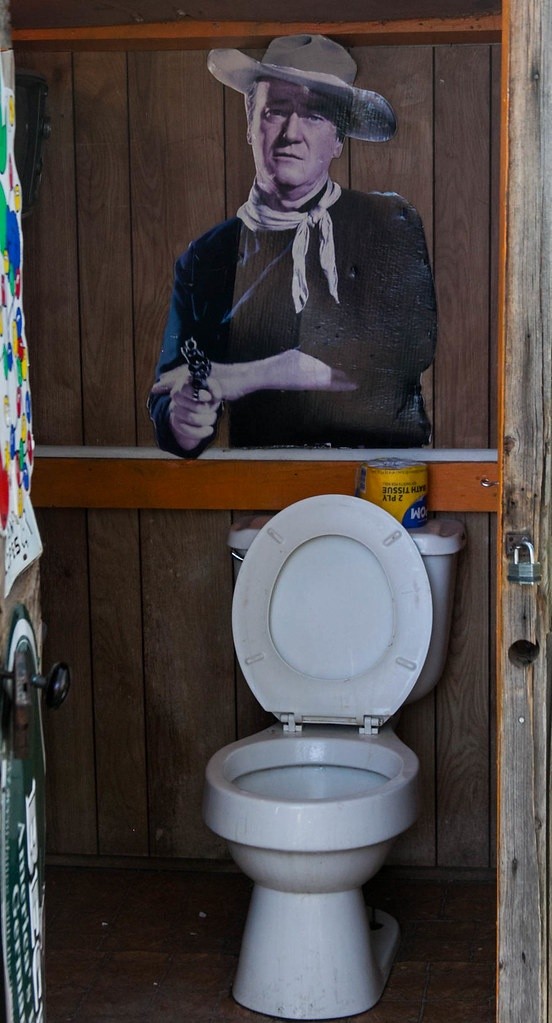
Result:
[358,455,432,530]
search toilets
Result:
[200,510,467,1023]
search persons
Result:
[144,32,440,459]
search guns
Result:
[180,336,212,404]
